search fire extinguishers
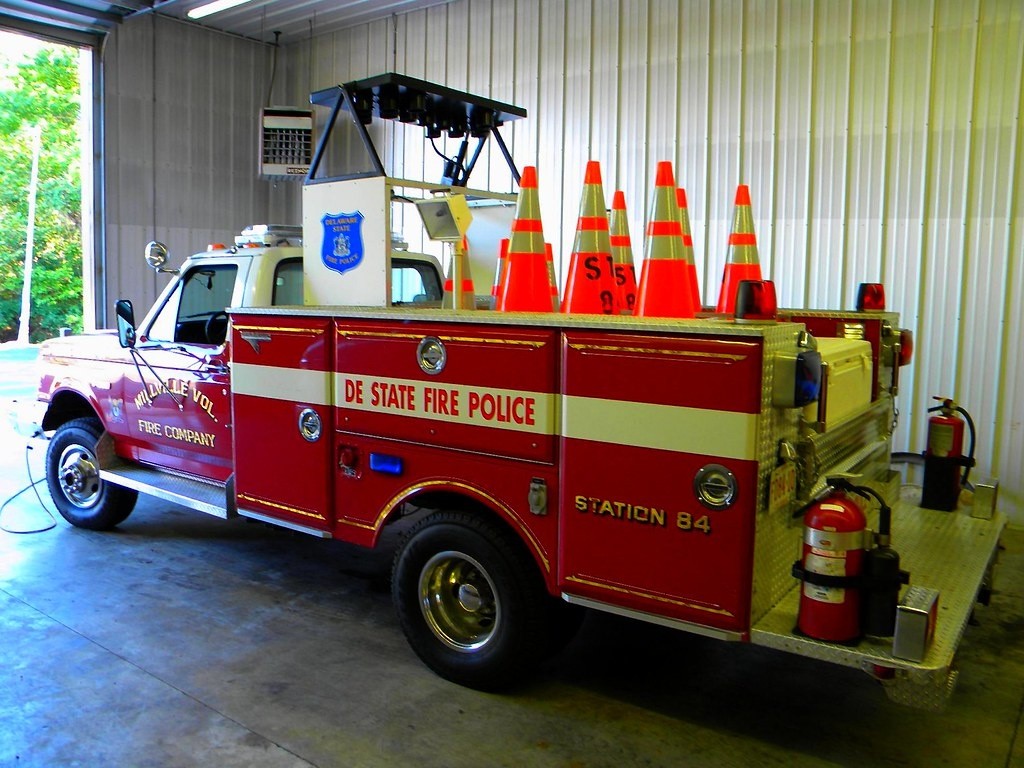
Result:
[925,396,979,508]
[795,465,894,641]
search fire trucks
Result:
[34,70,1010,693]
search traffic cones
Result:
[543,242,562,313]
[624,161,698,321]
[608,188,640,317]
[696,184,764,319]
[558,159,627,318]
[442,233,478,312]
[676,186,711,318]
[492,166,557,313]
[490,237,512,311]
